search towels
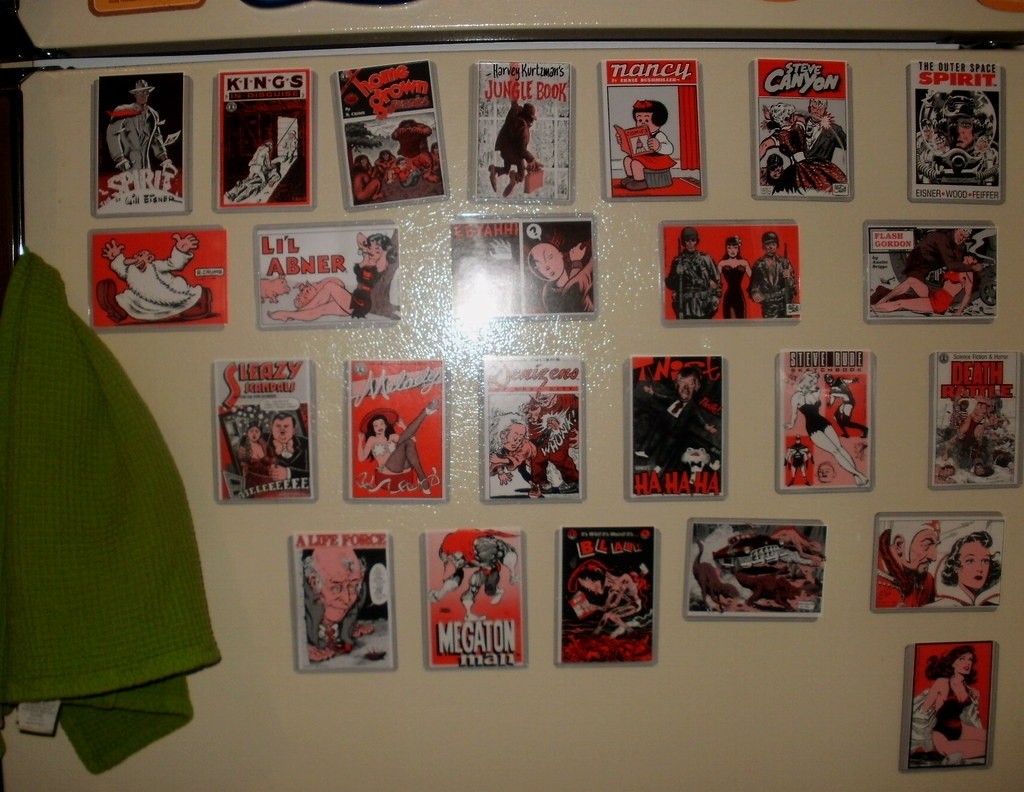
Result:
[0,251,222,776]
